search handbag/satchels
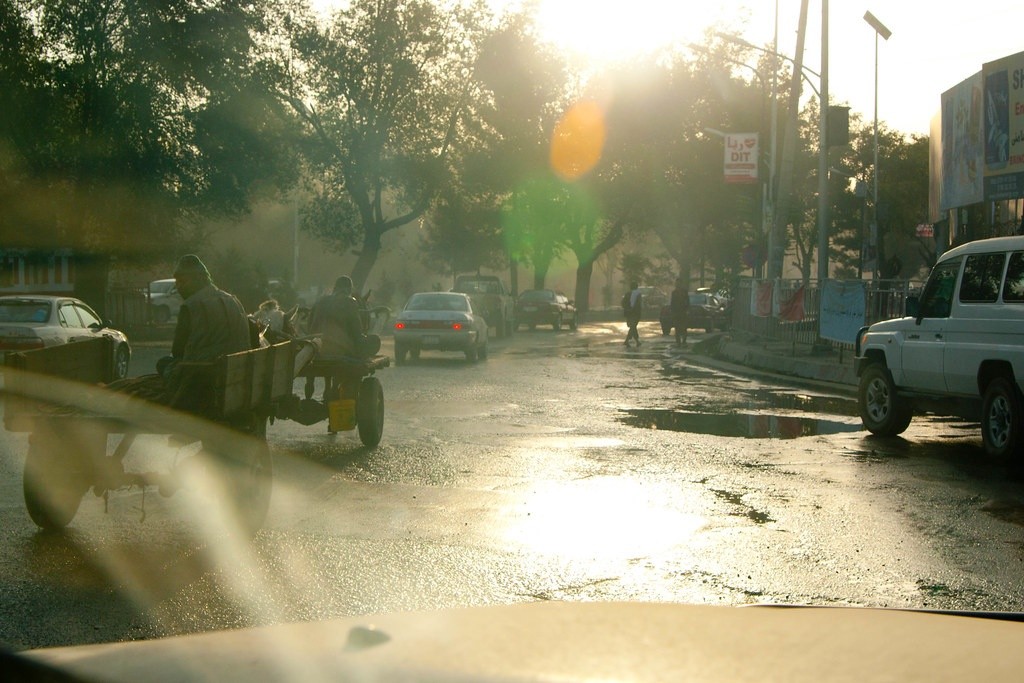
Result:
[620,295,626,308]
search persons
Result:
[304,276,363,400]
[671,271,688,348]
[32,254,252,445]
[621,281,642,347]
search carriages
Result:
[4,287,391,534]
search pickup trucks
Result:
[451,275,515,339]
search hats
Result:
[333,275,354,291]
[173,254,210,277]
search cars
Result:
[1,294,134,385]
[659,290,728,337]
[142,278,186,324]
[394,290,489,365]
[515,287,580,333]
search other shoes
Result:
[637,342,642,347]
[623,342,631,349]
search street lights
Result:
[715,0,835,354]
[686,0,810,337]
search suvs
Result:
[852,235,1024,466]
